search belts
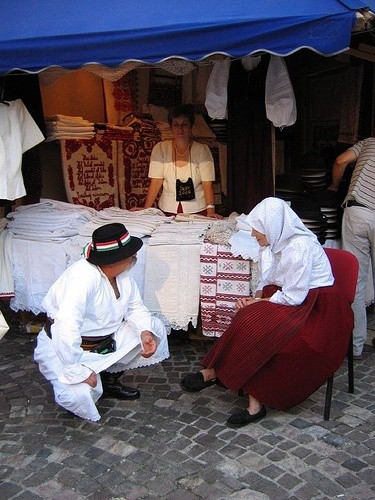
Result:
[342,199,367,209]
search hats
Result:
[82,224,143,264]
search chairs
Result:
[323,246,360,422]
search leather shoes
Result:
[105,381,141,400]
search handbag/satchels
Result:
[174,146,195,202]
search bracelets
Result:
[205,200,216,208]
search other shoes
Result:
[180,369,216,392]
[227,409,266,429]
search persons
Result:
[328,136,375,361]
[130,108,224,220]
[177,199,356,429]
[33,223,170,421]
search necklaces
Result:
[173,141,190,157]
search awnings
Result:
[0,0,375,76]
